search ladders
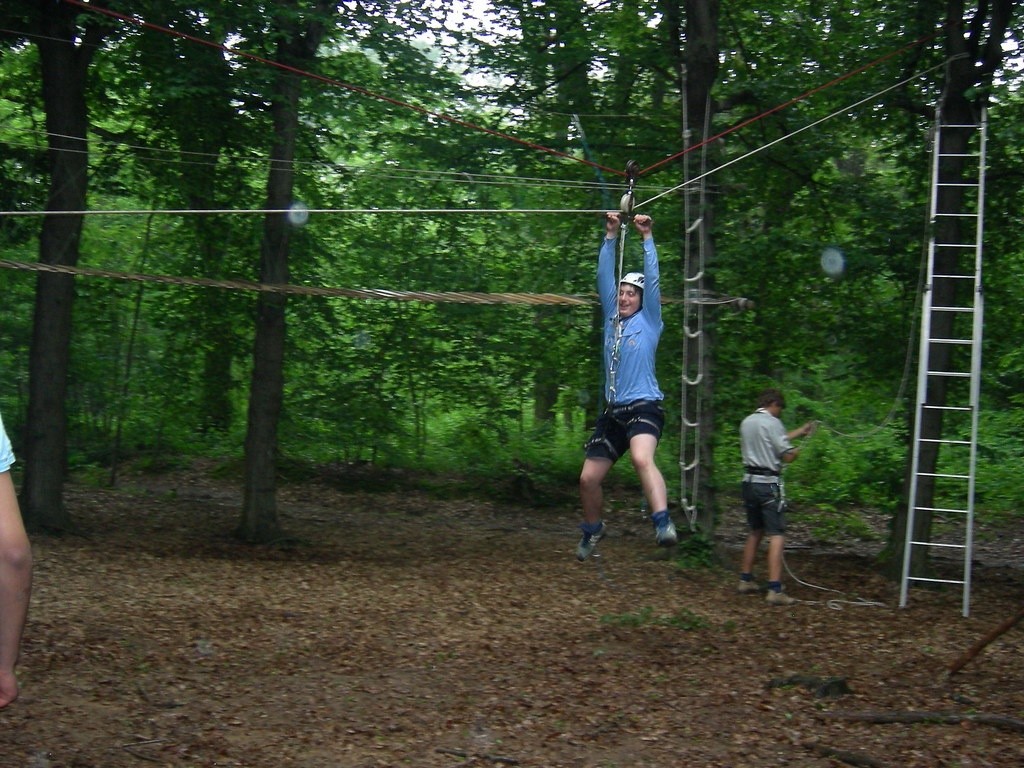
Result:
[896,104,990,616]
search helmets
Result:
[620,273,646,289]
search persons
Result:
[739,390,814,604]
[0,414,32,708]
[573,212,676,560]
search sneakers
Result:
[738,580,762,594]
[575,521,607,565]
[651,510,678,546]
[765,589,796,606]
